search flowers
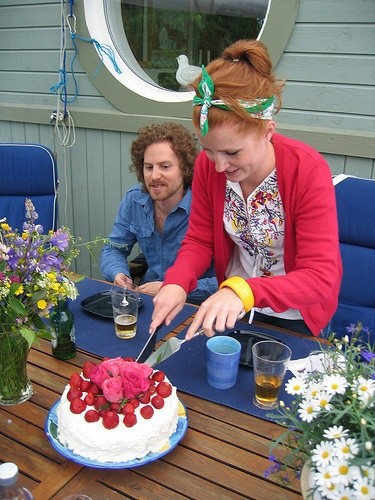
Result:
[0,198,129,400]
[89,358,152,413]
[264,321,375,500]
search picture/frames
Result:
[142,0,193,68]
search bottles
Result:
[50,295,76,360]
[0,461,33,500]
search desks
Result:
[0,270,332,500]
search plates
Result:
[80,290,144,318]
[46,398,188,468]
[225,330,288,369]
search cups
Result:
[110,283,139,339]
[252,340,292,410]
[206,336,242,389]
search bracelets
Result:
[219,276,254,313]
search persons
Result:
[100,122,218,305]
[147,39,343,341]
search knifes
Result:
[135,318,165,364]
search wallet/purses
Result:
[225,330,285,370]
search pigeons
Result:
[174,54,205,91]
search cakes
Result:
[57,356,179,463]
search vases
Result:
[0,327,34,406]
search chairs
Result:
[331,174,375,369]
[0,143,59,249]
